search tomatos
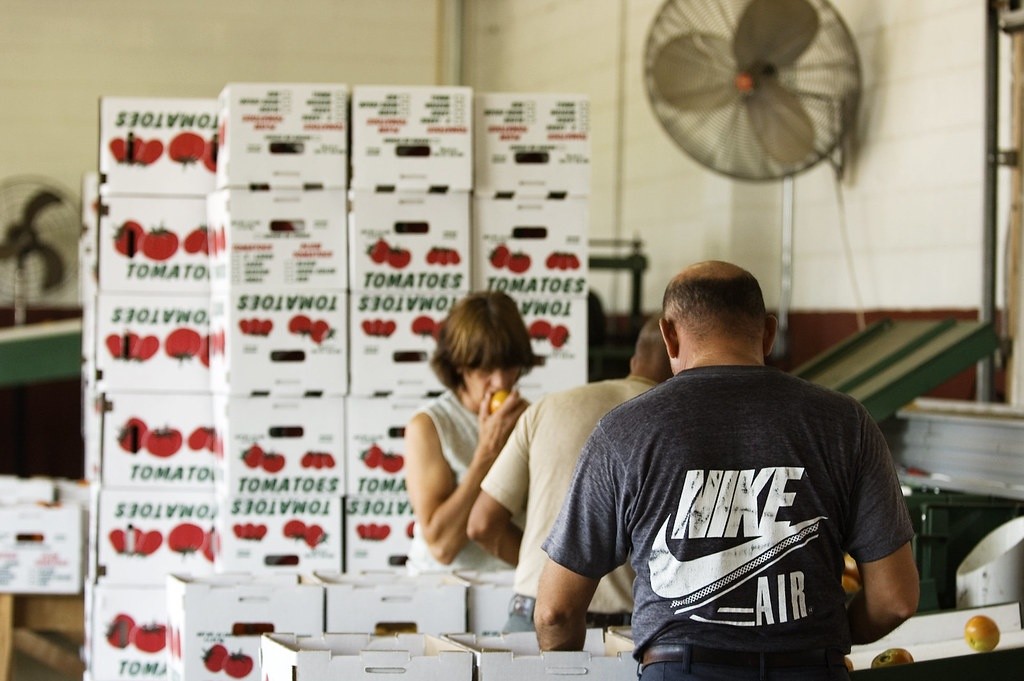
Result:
[870,648,913,670]
[965,616,999,651]
[489,391,509,413]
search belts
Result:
[641,644,848,673]
[513,597,625,628]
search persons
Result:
[467,311,675,634]
[403,292,534,575]
[534,261,920,681]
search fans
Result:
[642,0,862,183]
[0,174,82,326]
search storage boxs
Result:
[0,473,87,595]
[81,82,639,681]
